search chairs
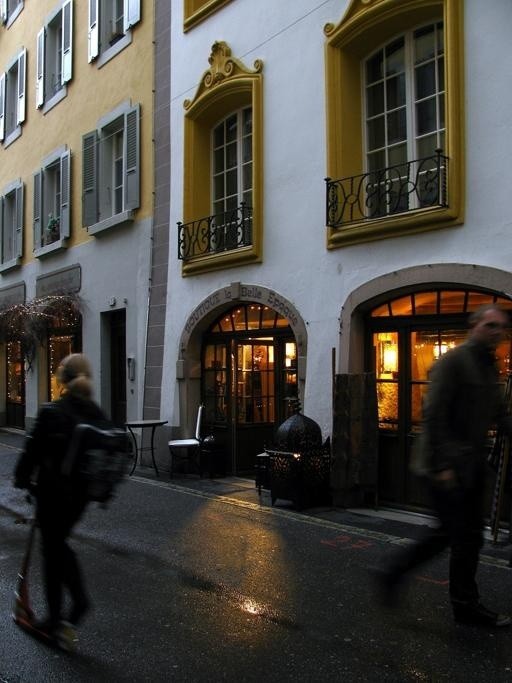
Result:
[167,402,206,480]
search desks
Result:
[122,418,169,479]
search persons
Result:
[368,301,510,630]
[13,354,110,627]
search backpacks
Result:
[70,421,132,502]
